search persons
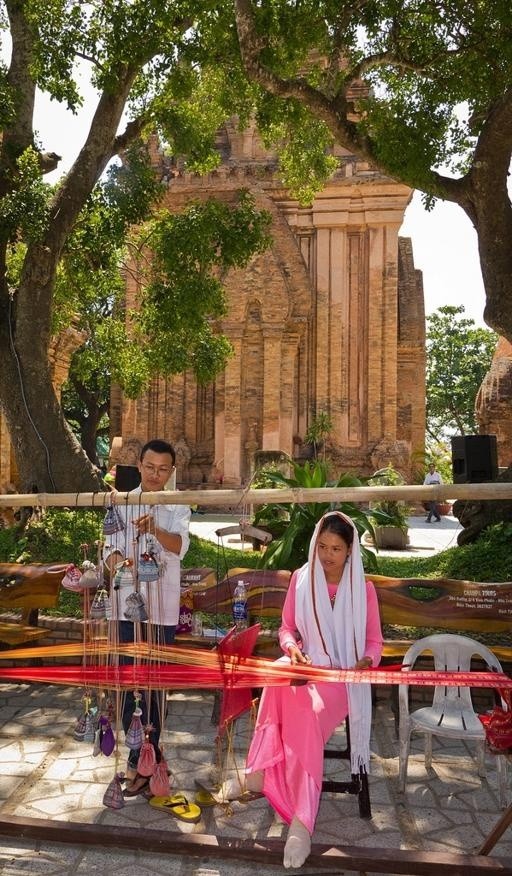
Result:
[421,463,441,523]
[102,438,192,797]
[243,510,386,869]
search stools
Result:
[315,713,375,821]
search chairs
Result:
[397,629,512,793]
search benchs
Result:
[0,561,511,665]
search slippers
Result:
[195,789,221,807]
[148,794,203,823]
[121,764,171,801]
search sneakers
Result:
[425,517,441,524]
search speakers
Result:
[451,435,498,484]
[115,463,140,492]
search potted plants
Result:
[365,461,410,550]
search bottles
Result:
[231,580,248,633]
